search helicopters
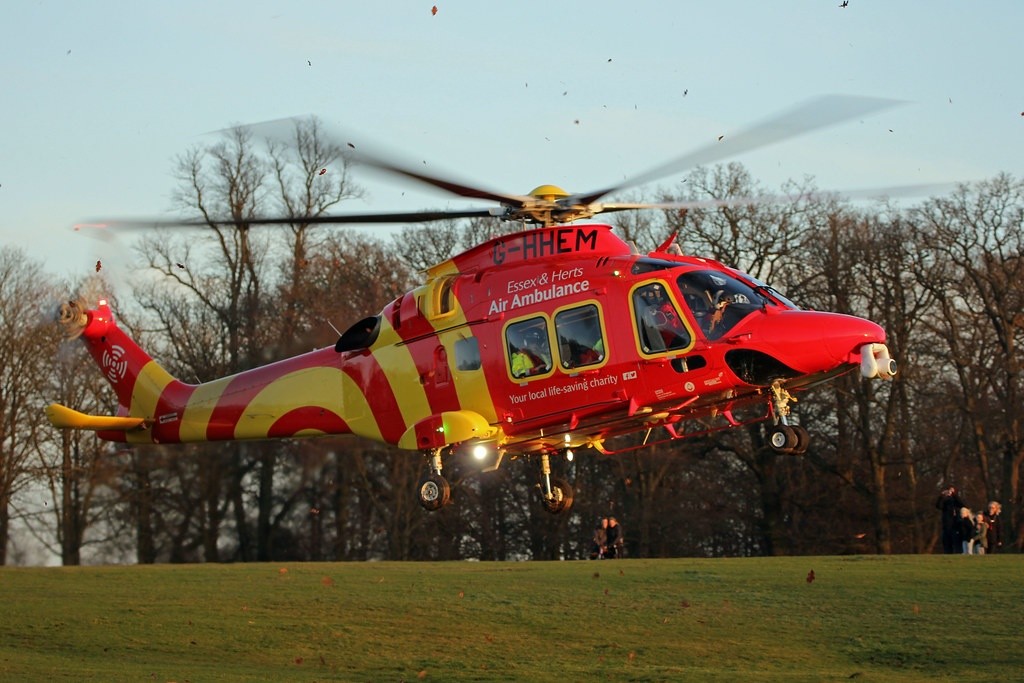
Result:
[36,85,964,515]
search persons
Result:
[970,512,987,554]
[937,484,960,555]
[607,517,624,558]
[512,328,552,379]
[987,500,1003,555]
[959,506,976,554]
[592,516,608,559]
[655,281,737,351]
